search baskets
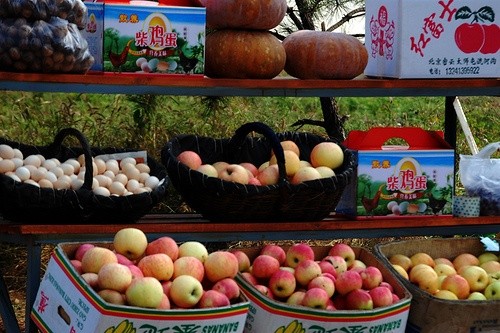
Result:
[0,128,169,226]
[162,122,354,224]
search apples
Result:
[178,139,344,185]
[64,228,242,310]
[389,250,500,302]
[234,242,399,310]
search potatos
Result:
[0,0,96,76]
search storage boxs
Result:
[366,0,500,78]
[336,127,456,219]
[83,0,207,75]
[30,238,500,333]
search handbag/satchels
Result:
[459,141,500,215]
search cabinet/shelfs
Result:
[0,74,500,333]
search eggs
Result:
[388,201,427,214]
[0,144,160,196]
[136,57,177,72]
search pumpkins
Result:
[204,0,288,32]
[281,21,367,80]
[204,29,286,79]
